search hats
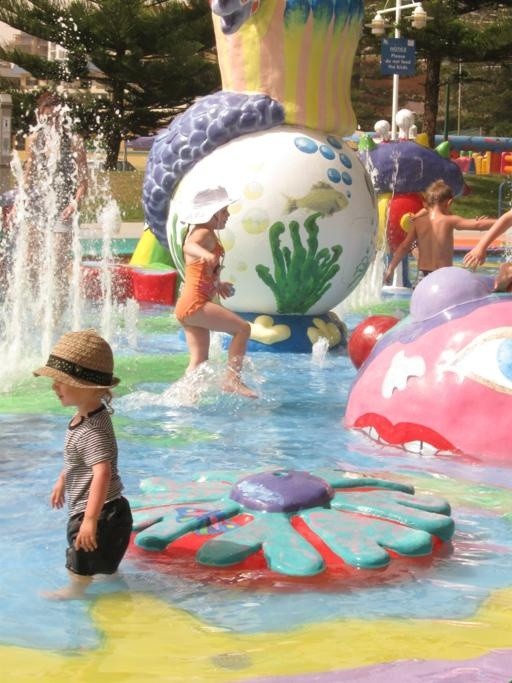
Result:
[180,186,235,226]
[33,330,121,391]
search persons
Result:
[409,208,487,259]
[33,330,134,600]
[462,211,512,292]
[5,90,88,323]
[385,178,497,292]
[175,185,261,399]
